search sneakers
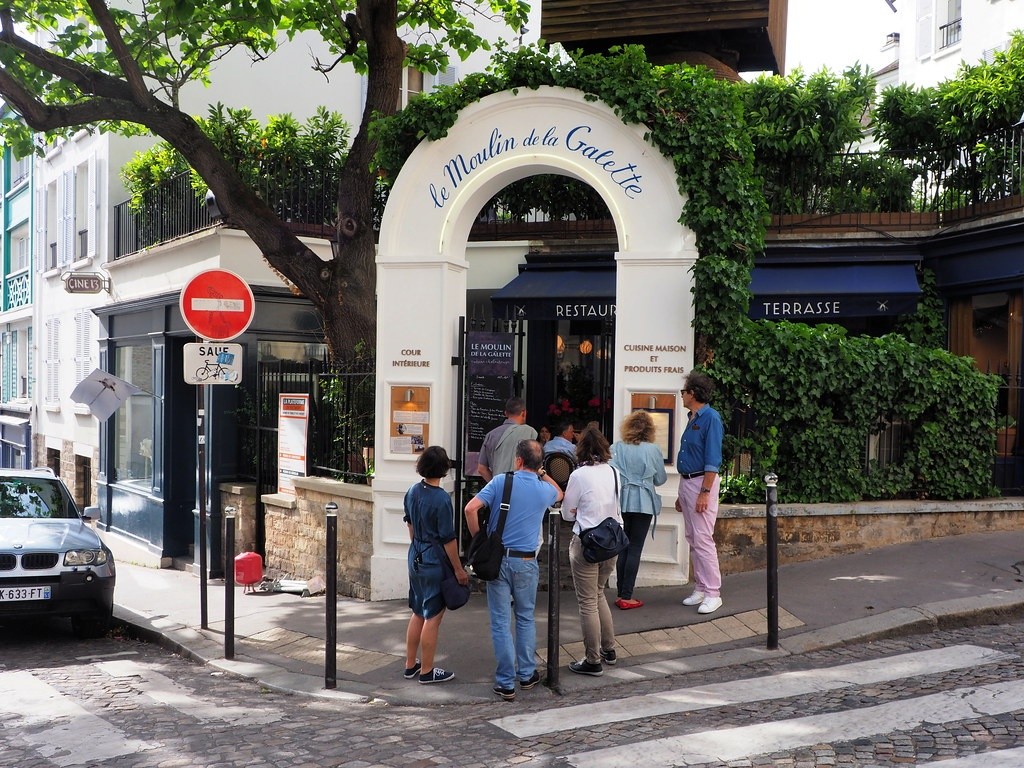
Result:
[599,646,617,665]
[519,670,541,690]
[493,686,516,698]
[403,658,422,678]
[698,596,723,614]
[683,590,705,605]
[569,657,603,676]
[419,667,455,684]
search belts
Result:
[502,549,536,558]
[682,472,705,479]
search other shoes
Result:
[615,597,644,610]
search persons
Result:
[478,398,537,483]
[540,425,551,445]
[464,439,564,700]
[674,370,723,613]
[544,423,581,465]
[403,446,470,683]
[609,410,668,609]
[560,428,623,676]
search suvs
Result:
[0,465,116,638]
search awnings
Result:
[491,264,919,320]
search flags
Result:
[70,368,141,423]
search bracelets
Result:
[539,470,546,478]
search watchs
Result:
[701,488,709,492]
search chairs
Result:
[0,487,32,513]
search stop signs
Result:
[179,268,255,341]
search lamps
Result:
[578,337,592,353]
[557,335,565,352]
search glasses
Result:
[680,390,690,395]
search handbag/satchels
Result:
[468,525,504,580]
[578,517,629,564]
